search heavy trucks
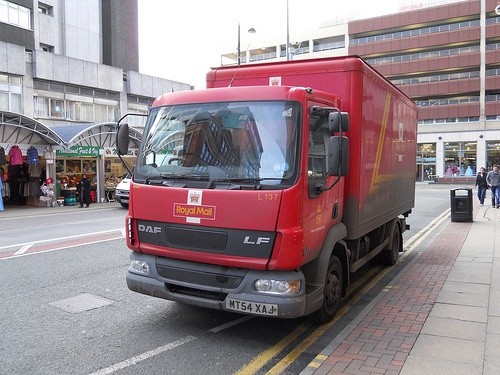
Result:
[125,54,419,326]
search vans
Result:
[115,170,132,209]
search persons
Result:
[465,166,473,176]
[459,165,465,174]
[453,166,458,174]
[486,164,500,209]
[445,164,453,175]
[475,168,488,206]
[429,168,434,181]
[75,174,92,208]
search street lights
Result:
[237,22,257,65]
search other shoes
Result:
[79,205,84,208]
[496,204,499,209]
[85,206,89,208]
[491,205,495,208]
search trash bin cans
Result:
[449,187,473,222]
